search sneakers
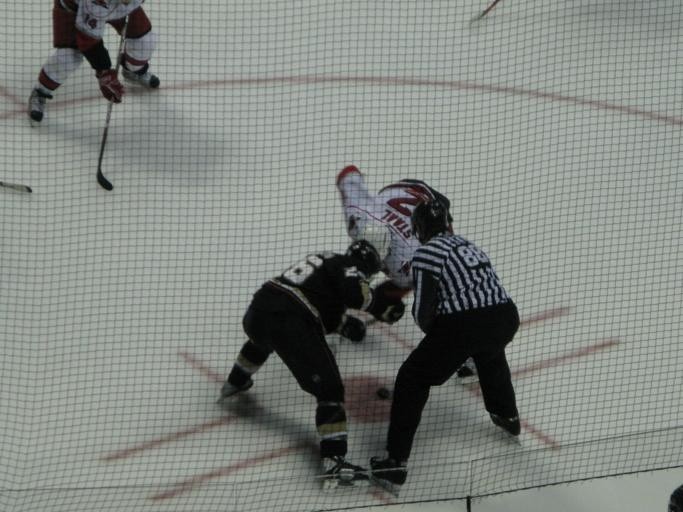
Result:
[370,456,407,497]
[321,456,370,491]
[27,88,46,127]
[217,378,253,402]
[490,410,520,444]
[122,64,160,89]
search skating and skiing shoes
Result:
[456,360,479,384]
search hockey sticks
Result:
[97,15,130,191]
[2,182,32,193]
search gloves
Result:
[342,316,365,342]
[381,299,405,322]
[96,68,125,103]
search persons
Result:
[367,197,522,485]
[335,164,452,323]
[26,0,160,121]
[218,236,406,479]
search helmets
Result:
[411,198,448,244]
[349,219,392,263]
[345,240,381,282]
